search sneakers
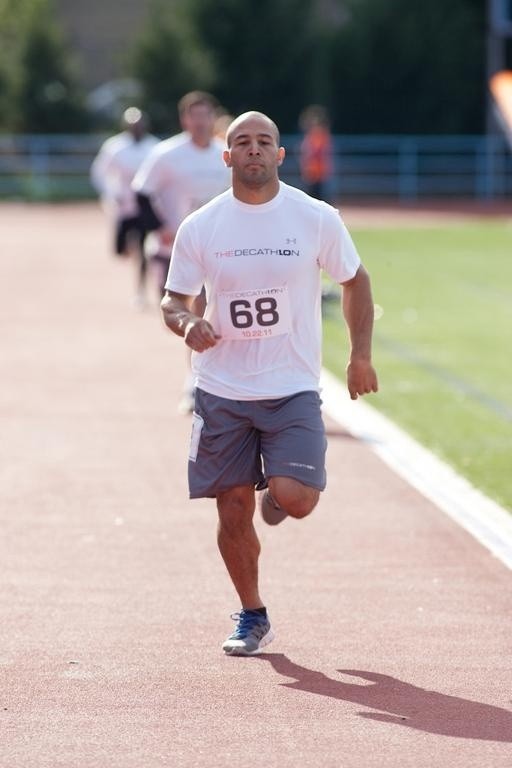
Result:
[222,607,275,656]
[261,489,287,527]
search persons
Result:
[298,103,333,201]
[88,88,237,414]
[160,110,381,655]
